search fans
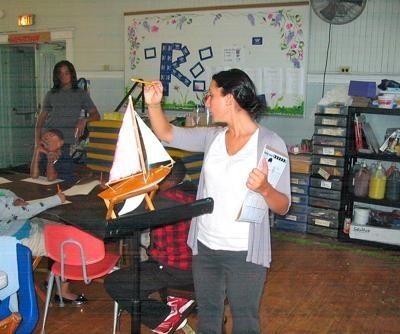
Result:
[310,0,367,26]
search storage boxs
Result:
[271,105,348,237]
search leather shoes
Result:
[54,294,87,305]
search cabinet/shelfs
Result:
[345,104,400,247]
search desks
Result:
[0,169,123,231]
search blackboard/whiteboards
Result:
[123,1,311,120]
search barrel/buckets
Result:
[353,205,371,225]
[348,159,400,202]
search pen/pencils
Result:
[131,78,156,86]
[56,183,60,192]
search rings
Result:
[252,178,257,185]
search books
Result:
[237,142,288,225]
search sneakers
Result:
[167,295,196,310]
[152,310,188,334]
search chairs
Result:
[0,224,198,334]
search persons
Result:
[102,154,196,334]
[1,182,88,305]
[33,59,100,150]
[29,127,79,181]
[143,68,292,333]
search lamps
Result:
[17,14,35,26]
